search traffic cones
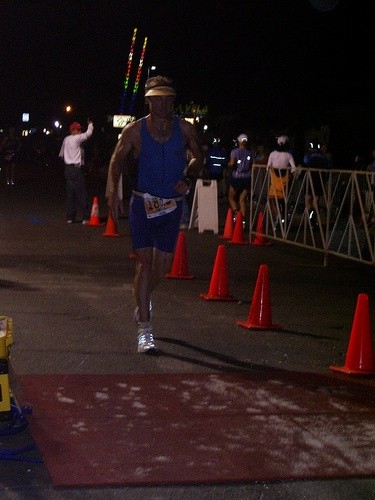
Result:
[199,244,239,302]
[164,233,195,280]
[248,212,273,245]
[83,196,104,226]
[103,207,122,237]
[219,208,234,241]
[328,293,375,376]
[235,264,283,331]
[229,211,250,245]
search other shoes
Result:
[135,330,156,352]
[136,306,153,326]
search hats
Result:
[145,85,176,97]
[278,136,287,144]
[237,134,248,142]
[69,122,83,129]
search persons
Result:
[205,137,225,198]
[227,133,254,228]
[0,126,21,186]
[266,133,297,225]
[335,150,374,224]
[18,125,48,160]
[302,142,329,224]
[60,117,95,223]
[106,75,202,353]
